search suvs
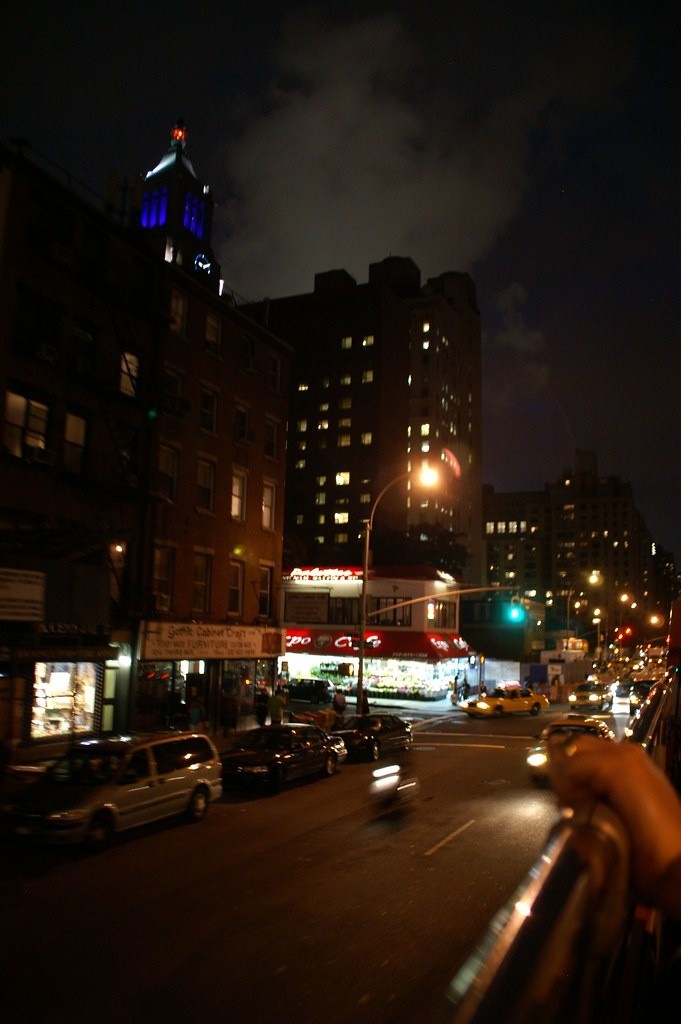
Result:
[4,728,226,857]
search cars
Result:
[225,723,349,791]
[607,654,668,715]
[331,712,416,761]
[460,681,550,720]
[567,675,614,712]
[526,711,617,786]
[276,676,337,703]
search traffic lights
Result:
[469,654,476,669]
[480,656,485,664]
[508,605,521,621]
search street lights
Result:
[564,571,604,650]
[618,592,630,657]
[355,468,439,715]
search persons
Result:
[453,676,470,706]
[549,735,681,914]
[480,681,487,693]
[522,675,560,697]
[333,690,346,716]
[255,689,287,727]
[356,689,370,715]
